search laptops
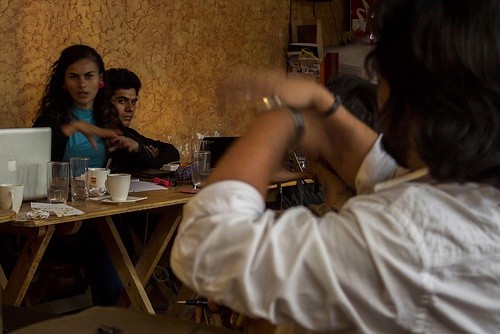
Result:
[0,127,52,201]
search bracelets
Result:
[317,93,342,118]
[253,95,305,145]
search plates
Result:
[101,196,148,203]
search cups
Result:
[0,184,24,214]
[88,168,110,194]
[107,173,131,201]
[191,140,212,189]
[47,162,69,205]
[70,157,90,203]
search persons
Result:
[170,0,500,334]
[32,44,181,315]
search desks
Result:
[0,179,315,317]
